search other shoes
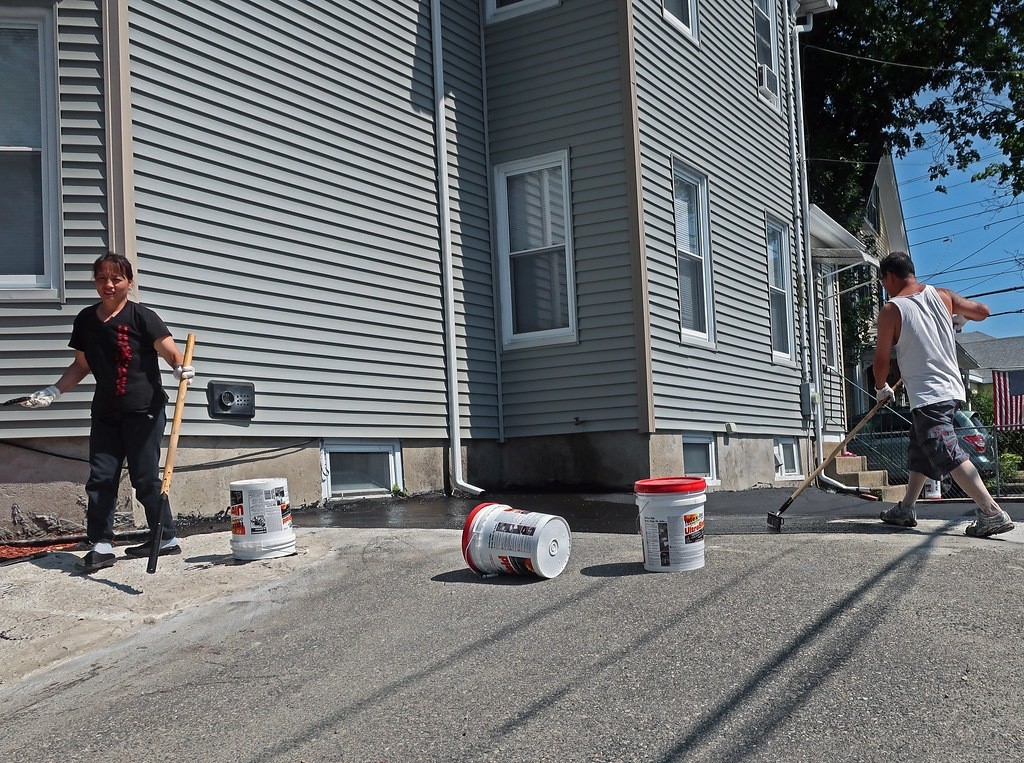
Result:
[73,551,116,572]
[125,540,181,557]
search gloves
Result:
[20,385,60,409]
[173,364,195,385]
[952,314,968,331]
[874,382,896,408]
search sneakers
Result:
[966,508,1015,538]
[880,500,917,526]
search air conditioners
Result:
[758,64,779,101]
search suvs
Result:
[845,406,1003,499]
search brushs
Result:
[3,397,33,406]
[766,378,904,528]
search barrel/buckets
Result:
[921,476,942,498]
[634,476,706,572]
[462,503,572,579]
[229,477,297,560]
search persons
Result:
[14,253,196,571]
[872,251,1016,537]
